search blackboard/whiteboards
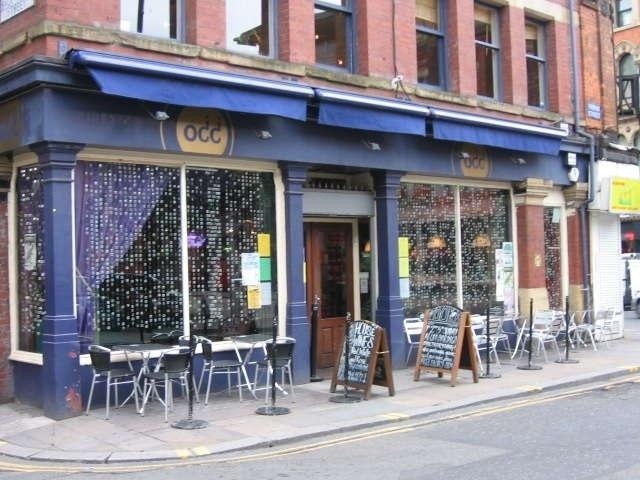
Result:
[332,320,382,390]
[372,327,392,387]
[416,305,467,374]
[459,313,482,371]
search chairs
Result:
[400,306,617,372]
[79,330,297,424]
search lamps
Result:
[509,152,528,166]
[361,135,382,151]
[149,110,170,122]
[455,142,471,159]
[252,128,272,140]
